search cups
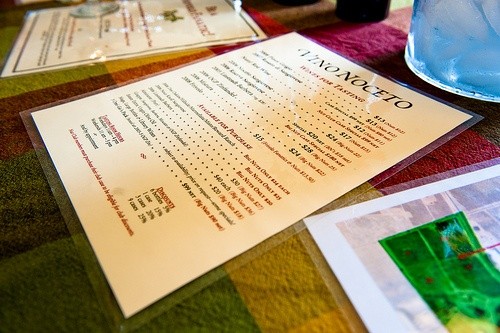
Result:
[335,0,392,23]
[404,0,500,102]
